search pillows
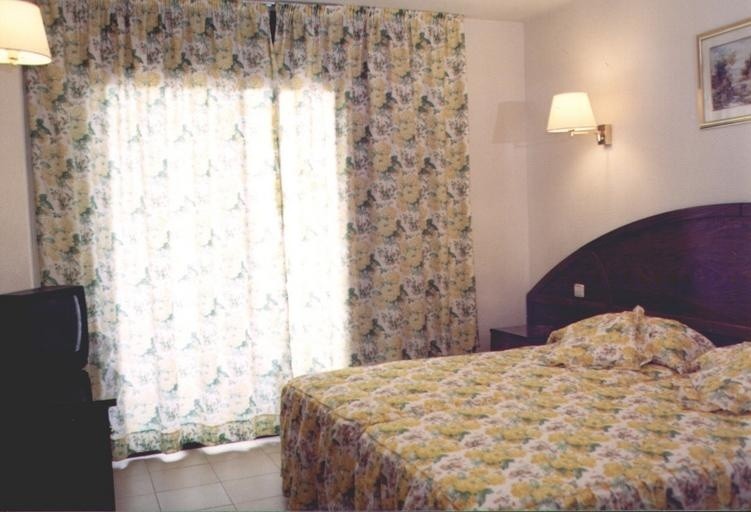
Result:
[680,341,751,412]
[527,304,717,376]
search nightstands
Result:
[490,324,547,351]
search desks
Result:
[0,365,117,512]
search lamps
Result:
[0,0,52,66]
[546,92,612,145]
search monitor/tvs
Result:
[0,286,89,387]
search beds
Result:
[279,201,751,512]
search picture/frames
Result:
[695,16,751,130]
